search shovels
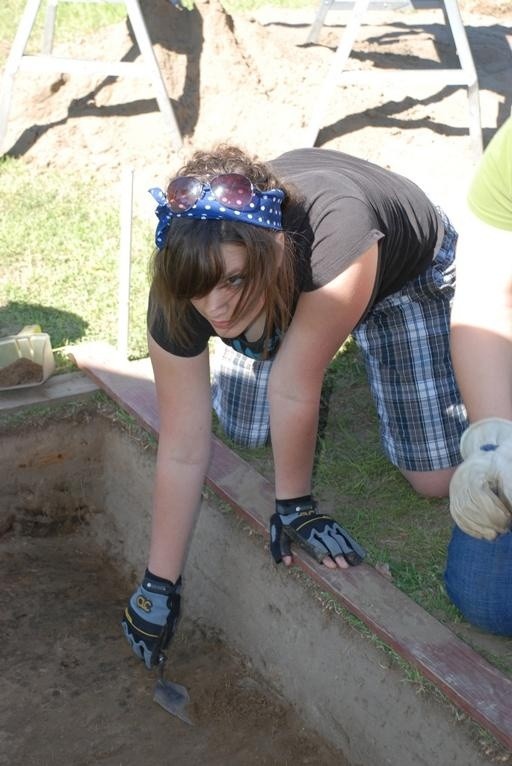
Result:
[152,664,199,727]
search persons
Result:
[120,140,472,673]
[445,108,512,642]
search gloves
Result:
[122,569,181,669]
[449,418,512,541]
[271,495,366,566]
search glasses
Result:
[167,174,254,214]
